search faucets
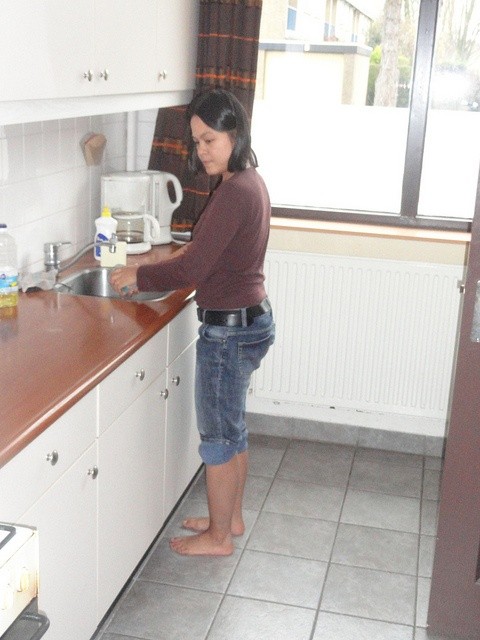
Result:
[42,241,116,278]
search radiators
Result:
[248,251,466,420]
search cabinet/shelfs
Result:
[0,386,97,639]
[427,165,480,640]
[1,1,157,126]
[97,299,202,630]
[157,1,199,108]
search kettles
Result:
[137,170,183,246]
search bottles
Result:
[0,224,17,321]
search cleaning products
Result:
[94,207,118,261]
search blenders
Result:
[100,171,160,254]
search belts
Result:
[197,297,273,329]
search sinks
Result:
[53,264,178,303]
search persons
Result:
[107,88,276,557]
